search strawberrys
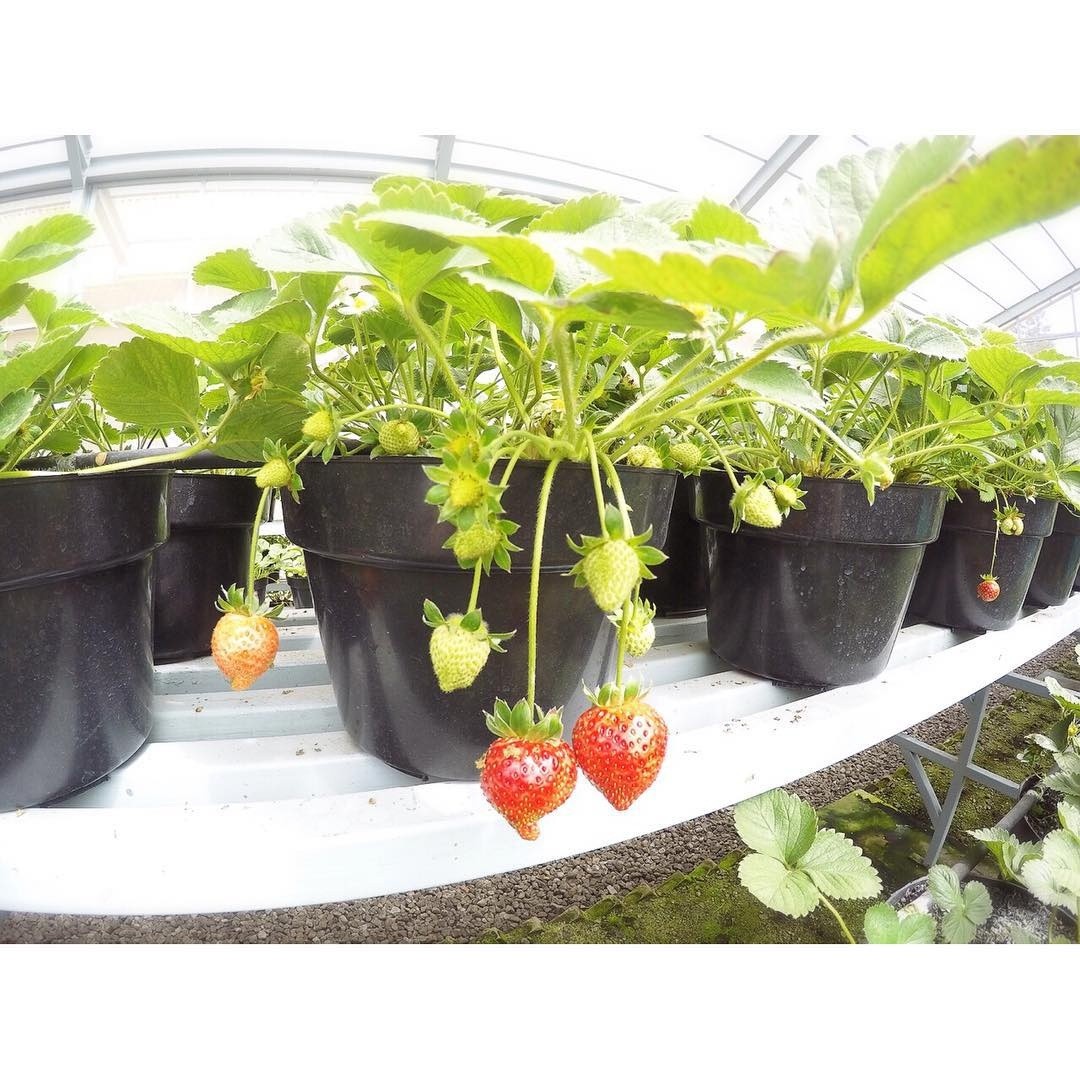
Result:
[257,389,897,533]
[607,596,656,660]
[421,408,525,577]
[478,677,577,841]
[976,573,1000,602]
[422,598,519,693]
[572,679,669,811]
[211,580,289,690]
[993,501,1025,536]
[560,503,670,617]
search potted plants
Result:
[0,132,1080,840]
